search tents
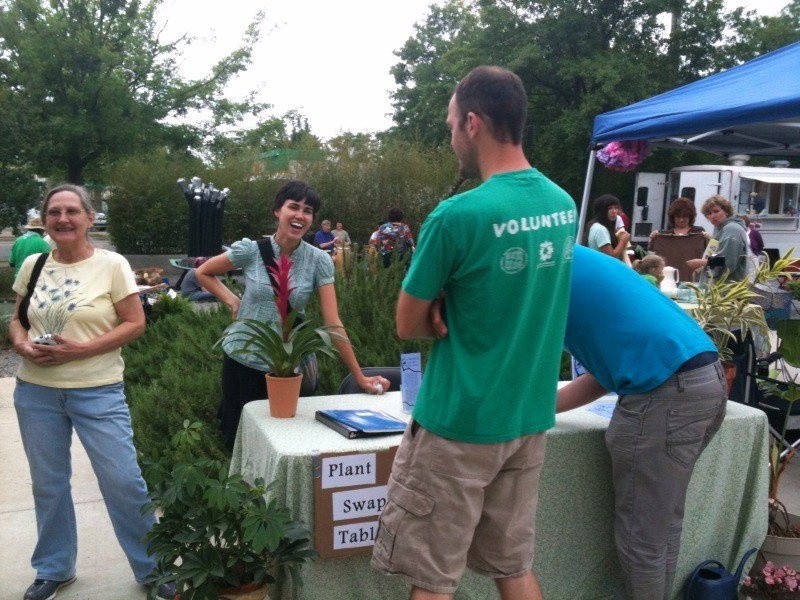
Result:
[569,38,800,380]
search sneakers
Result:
[145,573,179,600]
[23,574,77,600]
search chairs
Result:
[778,259,800,316]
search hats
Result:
[25,216,45,229]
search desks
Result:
[136,282,169,309]
[677,301,769,404]
[224,373,775,600]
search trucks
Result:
[630,164,799,274]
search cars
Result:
[24,203,109,233]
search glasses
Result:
[42,208,87,216]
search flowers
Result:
[738,559,800,600]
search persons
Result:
[748,192,765,215]
[738,214,765,258]
[374,207,416,269]
[685,193,751,300]
[368,64,580,599]
[179,256,220,304]
[7,214,52,282]
[330,220,352,255]
[137,271,169,299]
[367,221,387,249]
[314,218,342,258]
[582,194,631,262]
[554,245,728,600]
[613,214,625,231]
[647,196,710,283]
[192,179,391,455]
[6,183,185,600]
[42,234,57,251]
[616,203,630,234]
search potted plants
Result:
[139,418,322,600]
[753,369,800,448]
[686,244,800,401]
[739,318,800,572]
[207,253,362,419]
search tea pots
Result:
[660,266,680,298]
